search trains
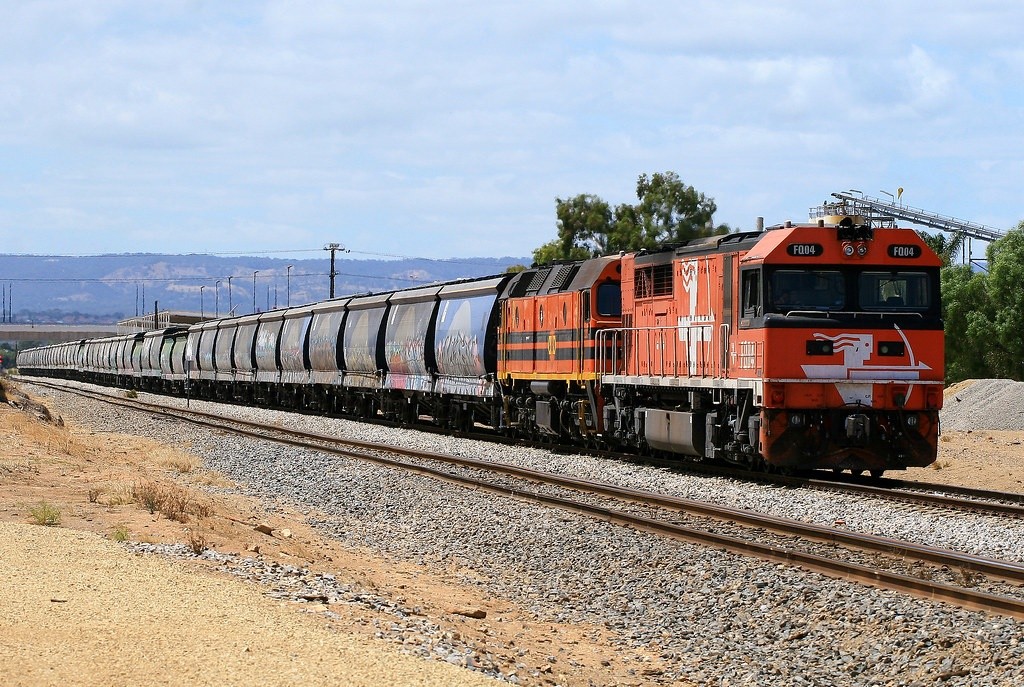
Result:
[16,217,945,478]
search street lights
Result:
[200,285,205,322]
[215,280,220,319]
[254,270,259,314]
[229,275,233,311]
[288,265,292,307]
